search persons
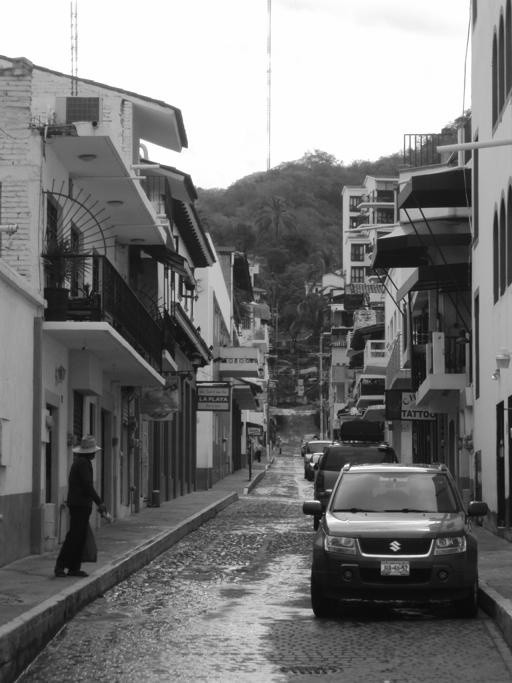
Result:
[55,434,107,578]
[256,442,262,462]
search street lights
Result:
[319,331,332,438]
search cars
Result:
[299,434,488,617]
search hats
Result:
[72,435,102,454]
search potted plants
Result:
[39,229,95,309]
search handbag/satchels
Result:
[79,520,97,564]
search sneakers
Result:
[55,566,92,577]
